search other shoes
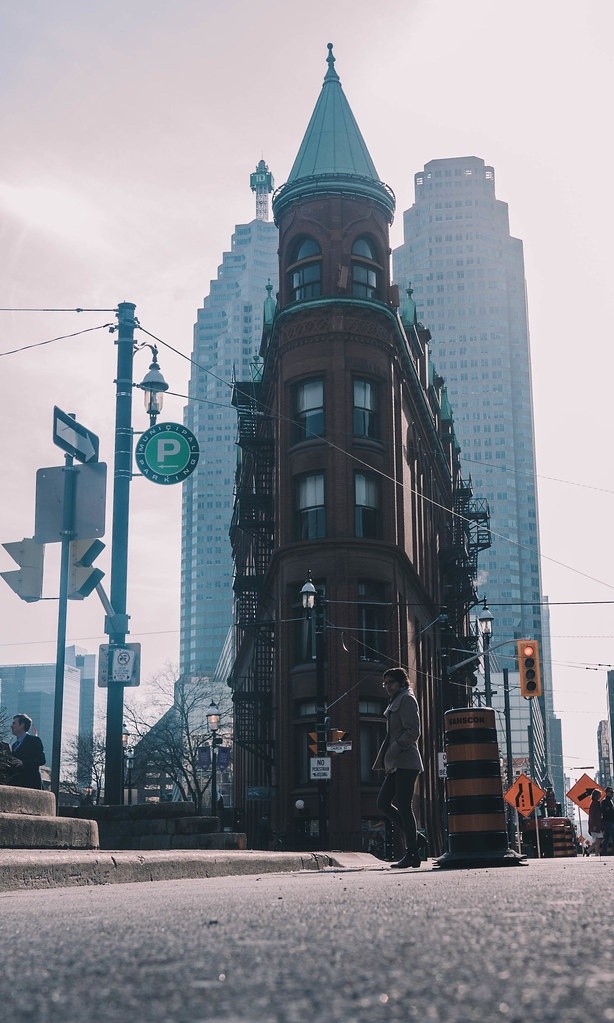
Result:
[390,851,420,869]
[585,849,590,857]
[417,832,429,848]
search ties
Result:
[12,741,18,751]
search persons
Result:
[583,789,603,855]
[0,742,11,785]
[371,667,428,868]
[9,714,46,788]
[601,787,614,849]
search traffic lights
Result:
[1,537,45,603]
[517,639,542,698]
[331,729,350,742]
[67,536,106,598]
[307,733,317,755]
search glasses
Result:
[382,681,398,687]
[608,790,614,793]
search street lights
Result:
[122,724,138,806]
[478,594,495,705]
[205,698,221,816]
[300,568,331,850]
[103,342,170,807]
